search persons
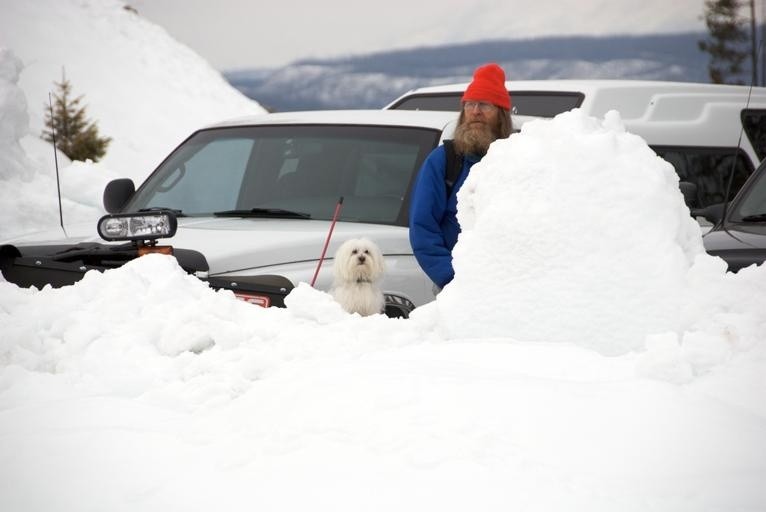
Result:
[409,63,513,290]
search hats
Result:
[460,63,513,112]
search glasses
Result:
[465,101,493,110]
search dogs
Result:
[327,237,386,317]
